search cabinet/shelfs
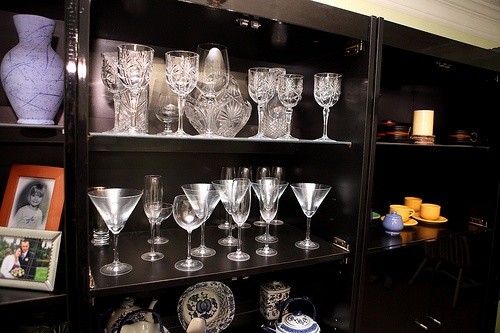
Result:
[0,0,500,333]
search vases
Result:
[0,14,65,124]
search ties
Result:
[22,253,25,261]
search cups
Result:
[388,204,415,223]
[404,197,423,212]
[260,281,291,320]
[419,203,441,221]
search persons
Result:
[14,183,45,228]
[0,239,36,280]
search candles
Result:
[413,109,434,136]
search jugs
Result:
[382,211,404,234]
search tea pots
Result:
[258,297,321,333]
[104,296,164,333]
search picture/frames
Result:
[1,162,65,231]
[0,227,62,291]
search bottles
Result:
[0,14,64,125]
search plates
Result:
[380,215,419,226]
[412,212,448,224]
[178,280,235,333]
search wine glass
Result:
[87,166,334,277]
[313,73,343,141]
[98,41,303,141]
